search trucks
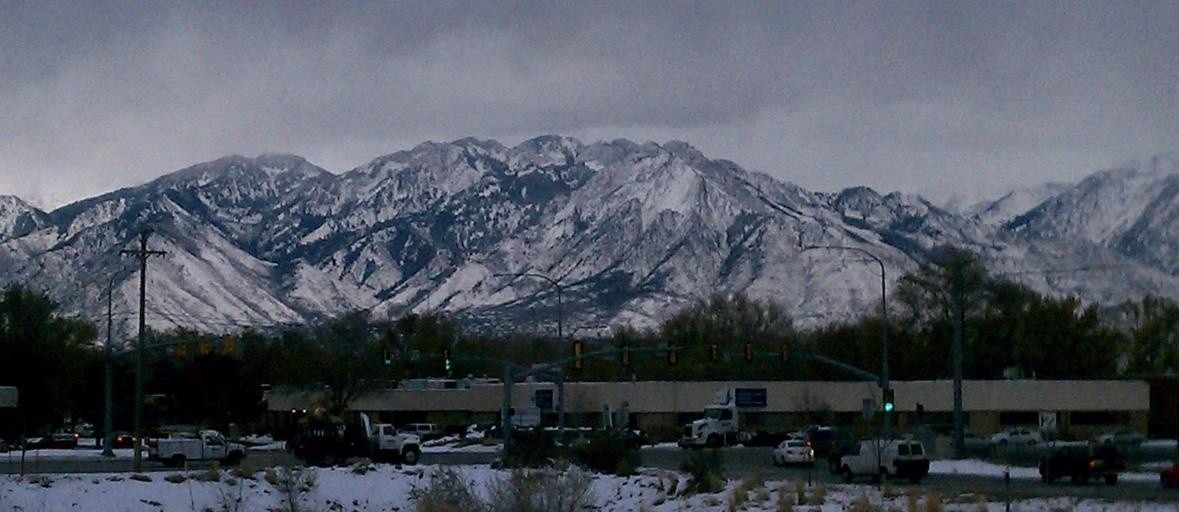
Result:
[680,401,811,449]
[359,411,422,465]
[146,430,247,470]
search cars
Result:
[771,439,815,467]
[23,427,79,450]
[1160,464,1179,488]
[986,426,1045,446]
[113,430,137,447]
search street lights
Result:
[101,267,140,459]
[493,271,566,462]
[804,243,891,434]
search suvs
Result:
[401,422,440,441]
[1037,438,1130,486]
[839,438,929,483]
[786,424,832,453]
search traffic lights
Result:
[384,350,391,364]
[444,357,450,371]
[883,388,895,415]
